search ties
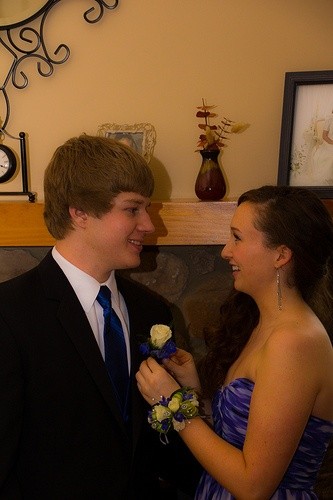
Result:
[95,284,130,387]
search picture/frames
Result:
[276,70,333,200]
[97,123,156,162]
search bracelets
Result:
[145,389,209,445]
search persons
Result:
[1,134,195,499]
[136,186,333,499]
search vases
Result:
[195,150,226,200]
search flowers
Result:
[142,324,176,363]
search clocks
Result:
[0,145,17,183]
